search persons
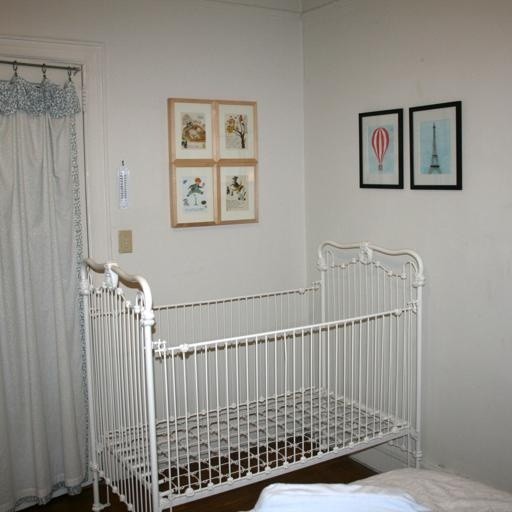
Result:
[186,177,205,197]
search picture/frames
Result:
[167,98,259,228]
[359,108,403,189]
[408,101,462,190]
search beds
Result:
[347,467,512,512]
[79,240,426,511]
